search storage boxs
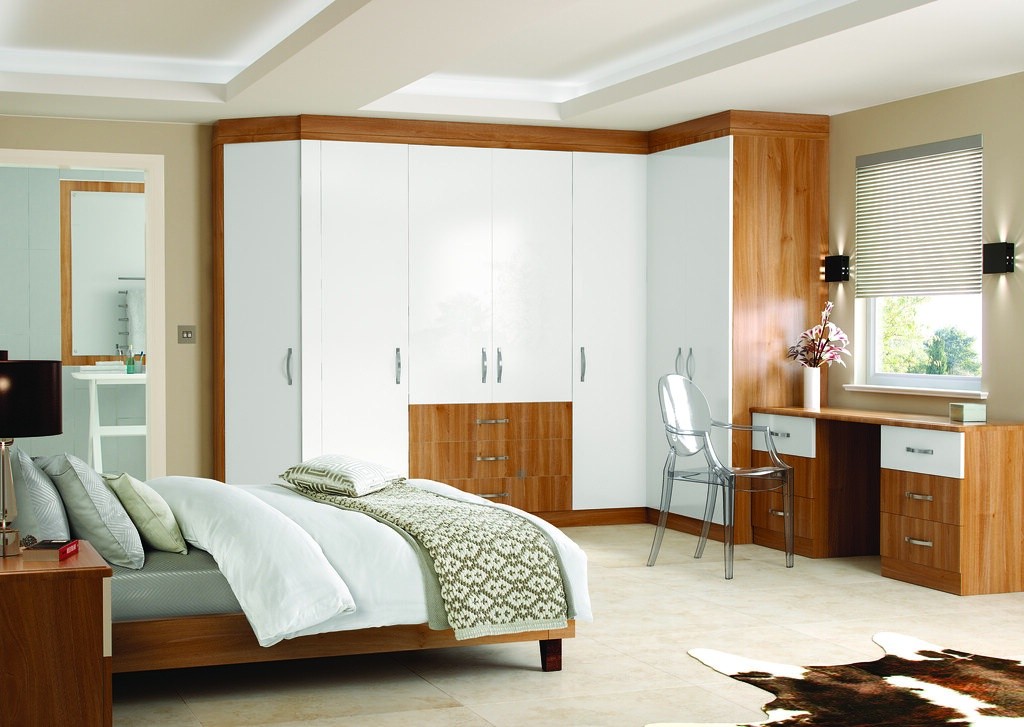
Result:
[949,402,987,423]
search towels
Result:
[125,286,146,355]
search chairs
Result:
[646,374,795,579]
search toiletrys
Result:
[127,345,135,374]
[114,344,121,355]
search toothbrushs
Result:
[139,351,144,362]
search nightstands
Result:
[0,539,114,727]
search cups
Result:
[134,361,143,374]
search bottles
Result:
[127,345,134,374]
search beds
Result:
[101,476,594,671]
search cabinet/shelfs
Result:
[573,127,646,528]
[408,120,572,529]
[301,114,408,480]
[212,117,301,484]
[646,109,831,545]
[750,407,1024,596]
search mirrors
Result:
[61,180,146,365]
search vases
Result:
[803,367,820,411]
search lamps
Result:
[825,255,849,282]
[983,242,1014,274]
[0,360,62,558]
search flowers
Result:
[786,300,852,368]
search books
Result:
[23,539,78,562]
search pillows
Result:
[102,471,187,556]
[9,445,69,541]
[32,452,144,570]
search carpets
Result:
[644,628,1024,727]
